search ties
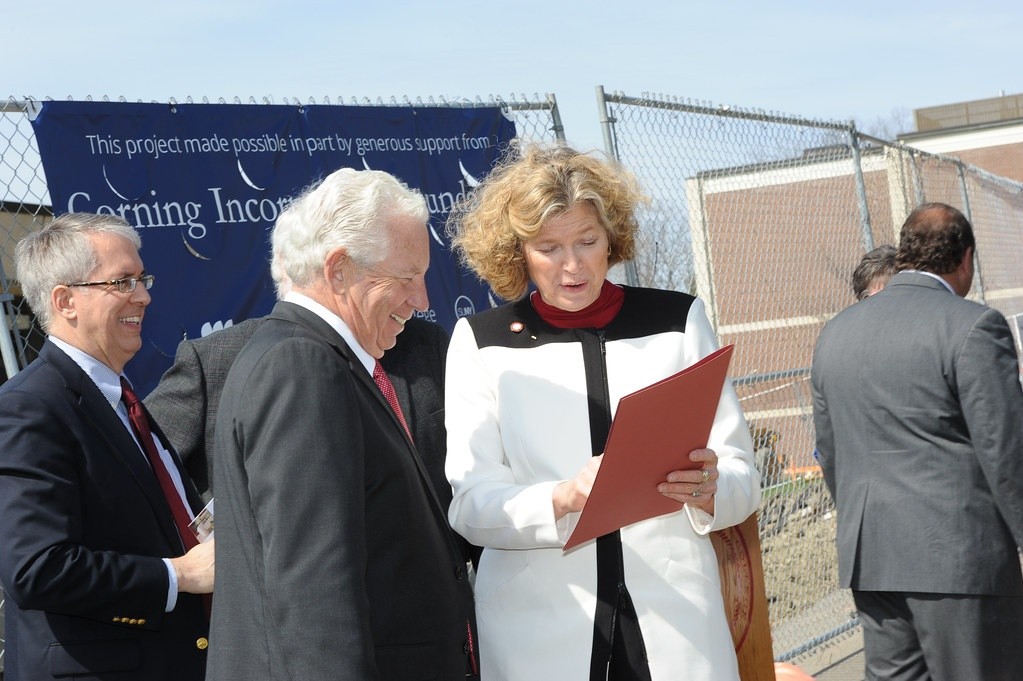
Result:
[374,357,413,450]
[119,376,198,555]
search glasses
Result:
[67,274,155,292]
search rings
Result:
[691,483,703,497]
[702,470,710,483]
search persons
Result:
[1,212,214,680]
[206,168,474,681]
[852,244,899,302]
[142,250,484,573]
[443,137,763,680]
[809,201,1022,680]
